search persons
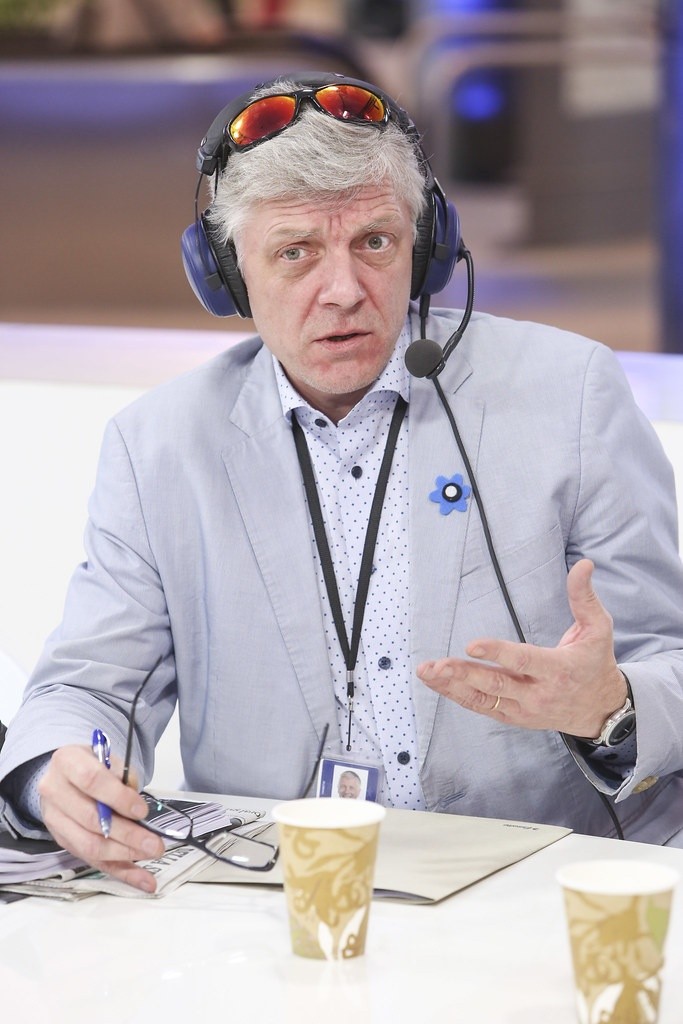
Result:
[0,72,683,894]
[337,772,361,799]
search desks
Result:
[0,788,683,1024]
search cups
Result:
[271,797,386,960]
[554,857,679,1023]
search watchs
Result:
[571,670,636,749]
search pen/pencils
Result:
[92,728,111,839]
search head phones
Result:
[182,70,461,319]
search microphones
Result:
[404,239,474,380]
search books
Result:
[186,808,573,897]
[1,799,275,902]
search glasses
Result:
[219,82,398,178]
[121,655,330,873]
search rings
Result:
[489,697,500,711]
